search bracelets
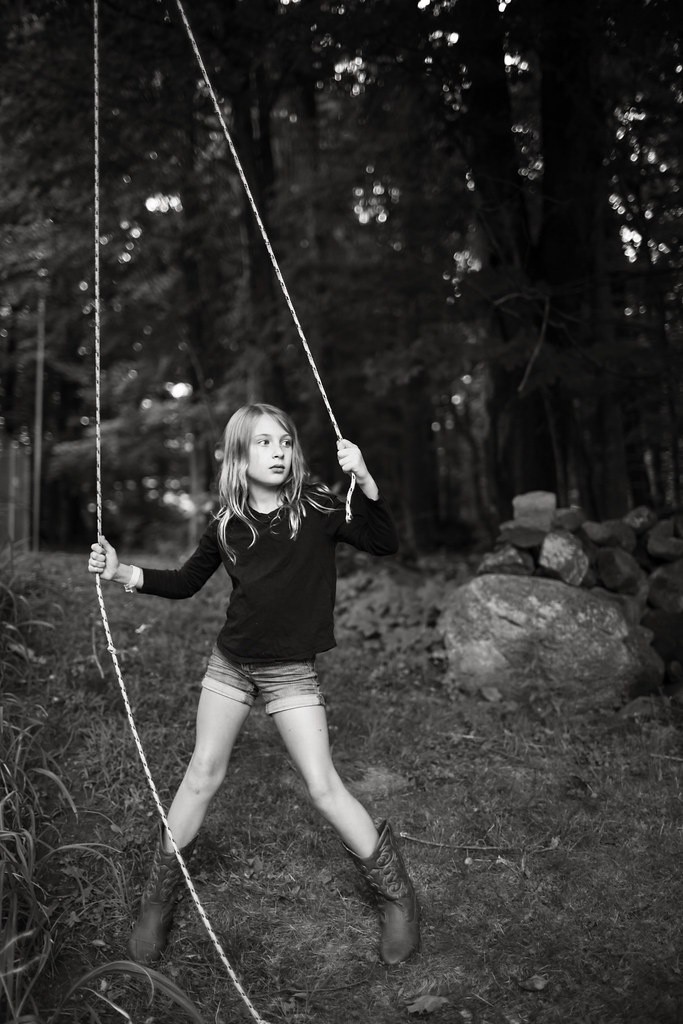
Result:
[122,563,142,595]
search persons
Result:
[88,400,423,971]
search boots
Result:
[128,819,200,968]
[340,816,421,966]
